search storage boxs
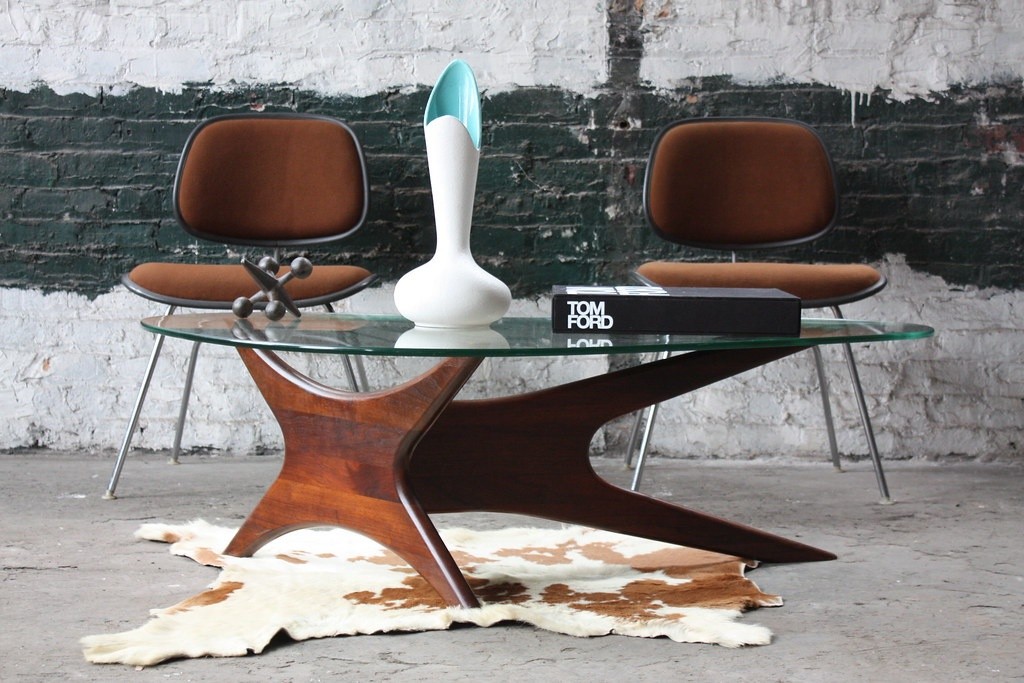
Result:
[551,284,803,336]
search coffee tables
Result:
[140,316,937,608]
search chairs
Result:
[626,116,897,506]
[101,111,381,501]
